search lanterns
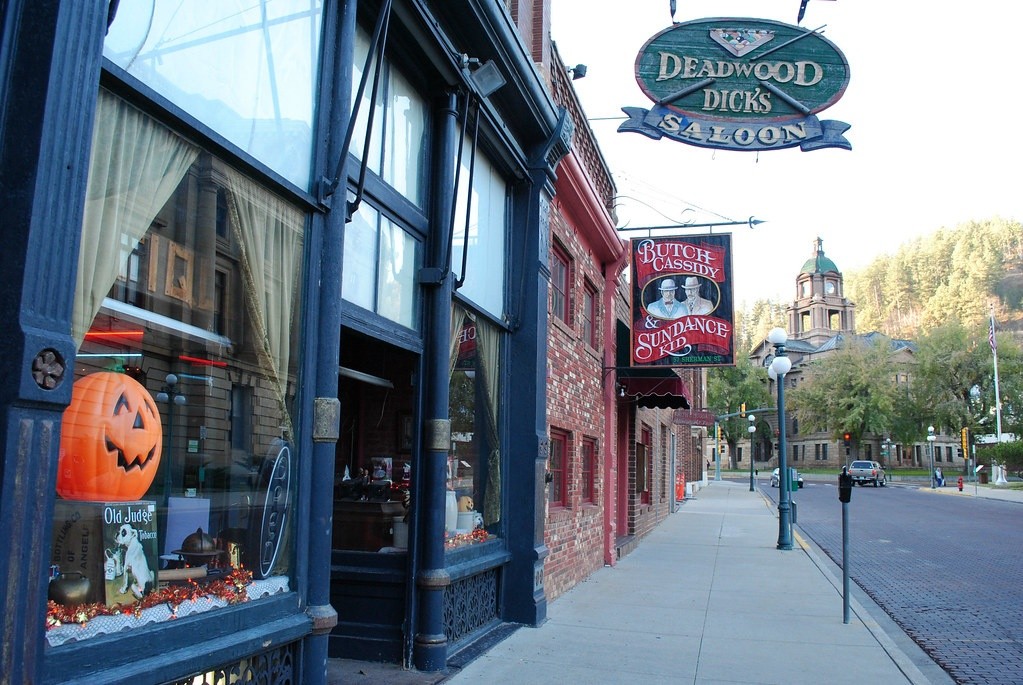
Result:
[56,355,162,501]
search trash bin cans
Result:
[980,472,988,484]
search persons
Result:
[935,467,944,487]
[707,460,710,470]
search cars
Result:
[770,467,803,489]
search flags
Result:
[989,313,997,354]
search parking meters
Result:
[838,466,853,624]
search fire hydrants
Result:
[956,476,964,492]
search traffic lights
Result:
[843,433,850,447]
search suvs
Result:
[848,460,888,487]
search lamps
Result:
[460,54,506,98]
[565,64,587,80]
[99,297,231,347]
[338,366,394,389]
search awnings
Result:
[618,319,694,410]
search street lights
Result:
[747,414,756,492]
[156,375,186,509]
[926,426,936,489]
[768,325,792,551]
[881,438,896,482]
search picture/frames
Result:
[396,408,413,454]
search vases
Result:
[446,491,458,532]
